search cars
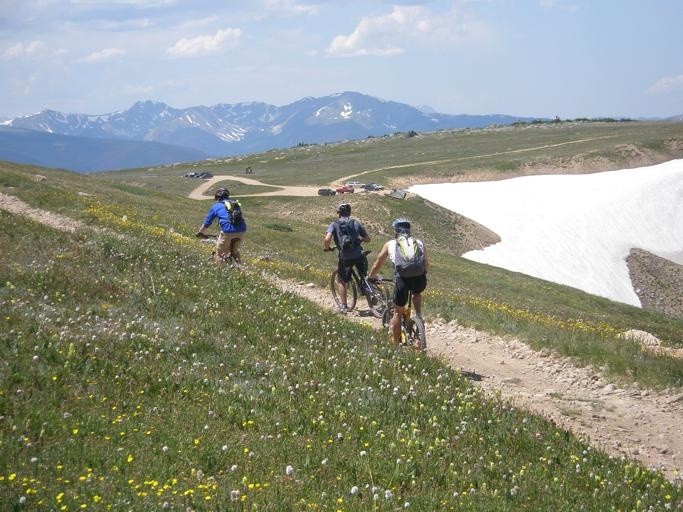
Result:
[182,173,213,180]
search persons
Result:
[196,188,246,259]
[324,204,371,314]
[369,219,428,343]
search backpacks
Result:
[336,219,356,252]
[218,199,242,225]
[395,233,424,278]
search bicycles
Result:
[360,275,425,353]
[197,230,244,271]
[323,241,391,317]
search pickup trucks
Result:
[336,185,355,194]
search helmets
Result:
[215,188,229,201]
[393,218,410,231]
[337,204,351,216]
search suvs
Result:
[344,181,385,191]
[317,189,336,196]
